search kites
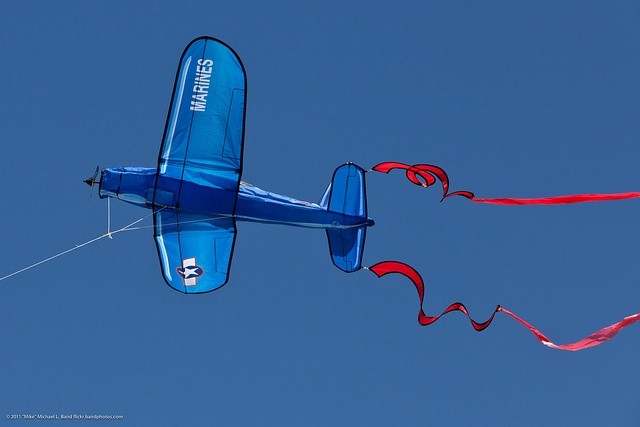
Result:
[83,33,640,351]
